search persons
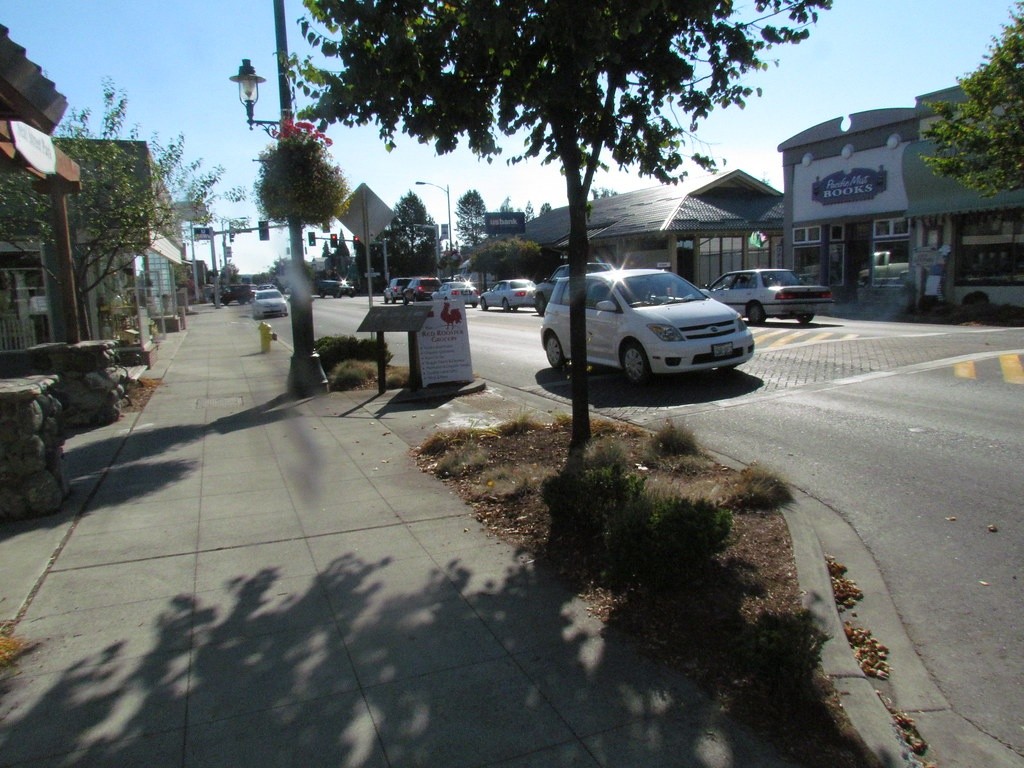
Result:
[737,277,748,287]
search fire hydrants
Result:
[257,322,279,353]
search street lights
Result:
[416,182,454,282]
[221,217,247,267]
[229,57,347,396]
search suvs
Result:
[221,284,257,305]
[382,278,412,304]
[402,277,442,305]
[535,263,615,317]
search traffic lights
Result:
[308,232,315,246]
[329,234,338,248]
[353,236,361,249]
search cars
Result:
[540,267,755,389]
[251,288,289,317]
[480,279,537,312]
[430,282,478,308]
[703,268,835,325]
[317,281,355,298]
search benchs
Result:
[115,353,148,407]
[856,288,904,321]
